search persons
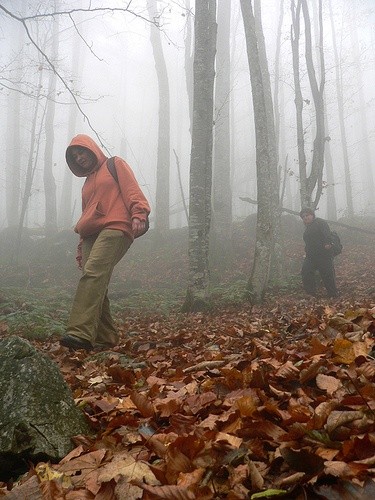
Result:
[300,207,338,298]
[60,134,151,352]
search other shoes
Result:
[59,333,94,352]
[93,342,111,352]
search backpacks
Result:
[315,217,343,256]
[106,156,150,239]
